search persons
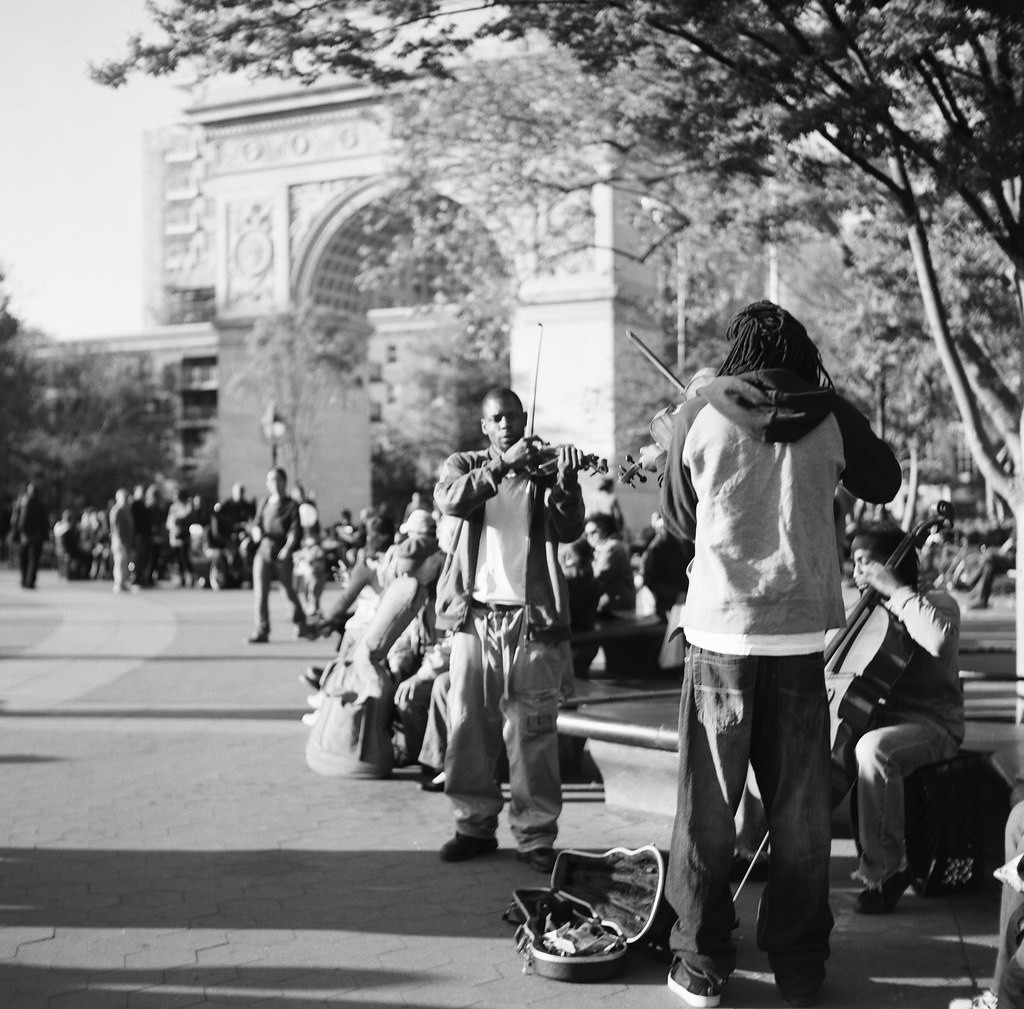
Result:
[440,389,587,873]
[670,300,904,1002]
[9,466,1024,1009]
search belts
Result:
[472,600,522,612]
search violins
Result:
[523,442,608,478]
[616,368,721,490]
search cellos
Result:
[733,499,956,904]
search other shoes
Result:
[952,581,971,593]
[965,598,988,610]
[307,665,323,680]
[856,865,916,913]
[946,990,998,1009]
[665,963,722,1008]
[248,632,268,643]
[419,771,445,793]
[299,674,320,692]
[307,693,324,708]
[301,709,319,728]
[439,832,498,860]
[730,853,770,881]
[516,848,554,874]
[297,619,309,637]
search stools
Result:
[851,753,1014,896]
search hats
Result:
[399,510,437,534]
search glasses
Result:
[584,527,598,536]
